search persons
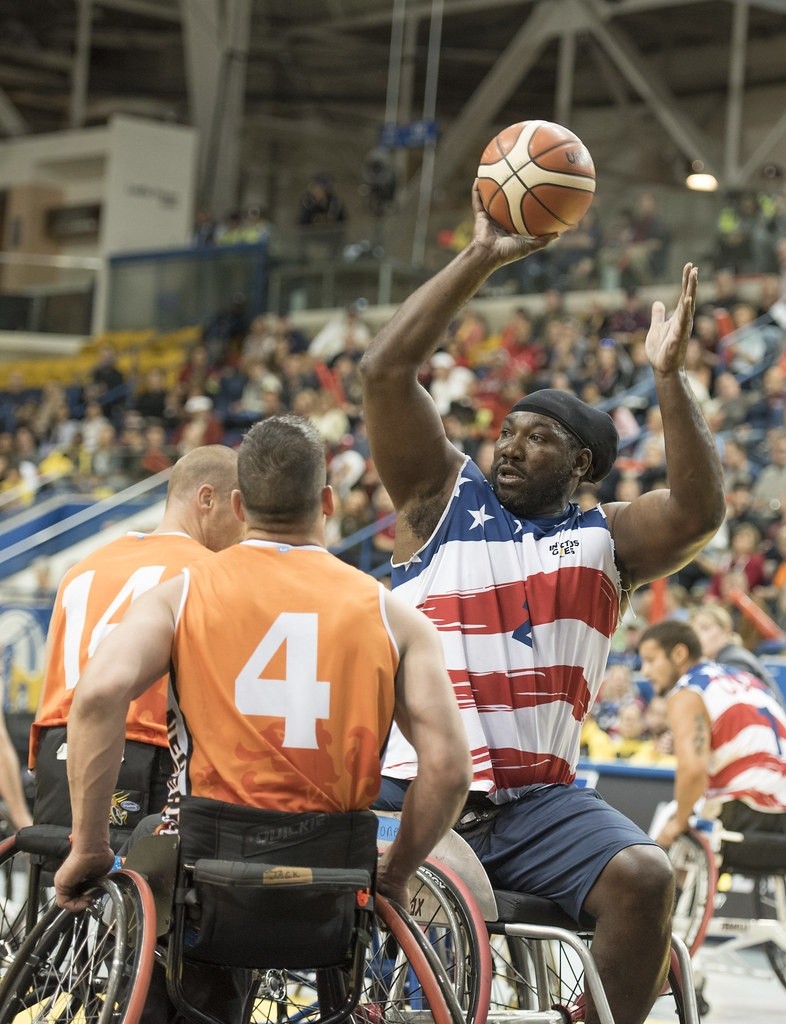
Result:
[0,174,786,754]
[0,709,33,833]
[358,177,727,1024]
[639,621,786,917]
[54,417,474,1024]
[28,444,245,770]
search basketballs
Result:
[476,118,598,236]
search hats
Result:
[510,389,619,482]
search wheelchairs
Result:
[656,798,786,997]
[0,725,702,1024]
